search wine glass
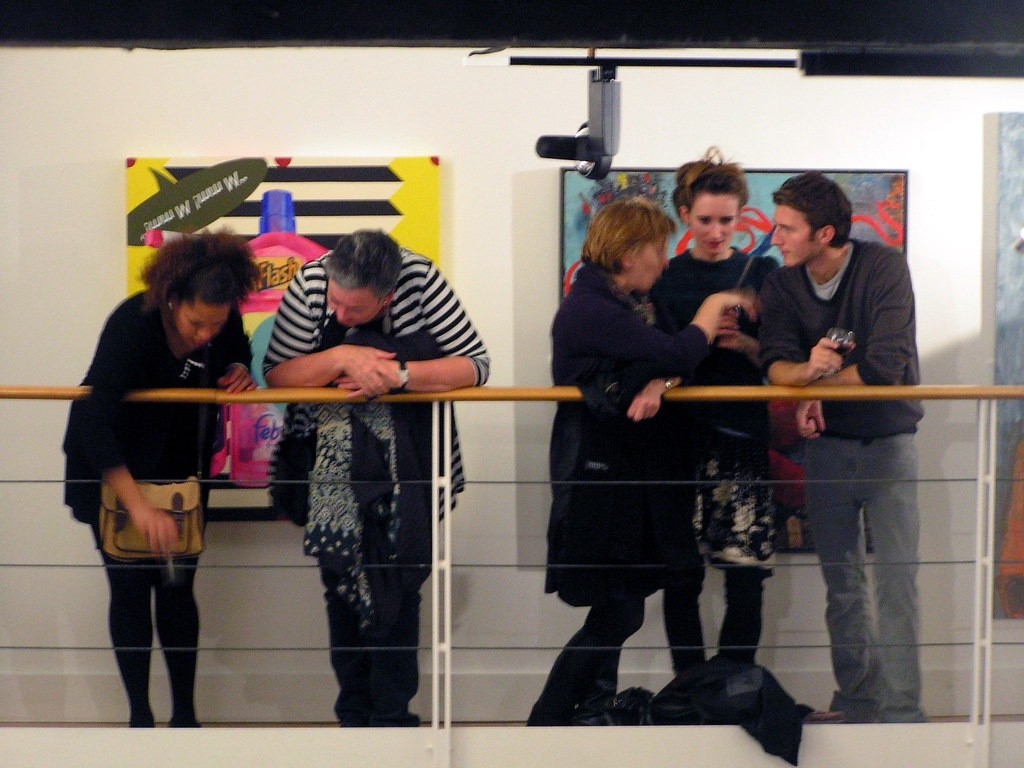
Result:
[817,327,854,381]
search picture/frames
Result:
[554,158,914,561]
[124,152,448,525]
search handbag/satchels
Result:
[97,480,205,559]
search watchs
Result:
[398,360,409,390]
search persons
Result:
[651,148,779,679]
[262,229,491,727]
[758,172,925,724]
[528,199,755,725]
[62,225,258,729]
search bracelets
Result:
[661,377,676,391]
